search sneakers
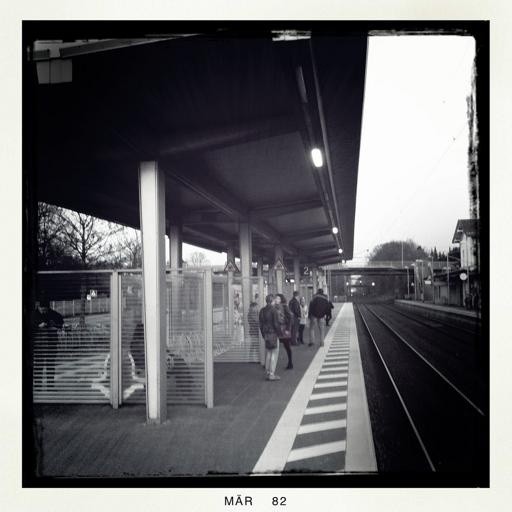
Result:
[264,370,282,381]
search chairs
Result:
[80,347,166,408]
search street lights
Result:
[404,230,471,306]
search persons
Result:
[36,299,64,392]
[247,288,334,381]
[129,322,202,399]
